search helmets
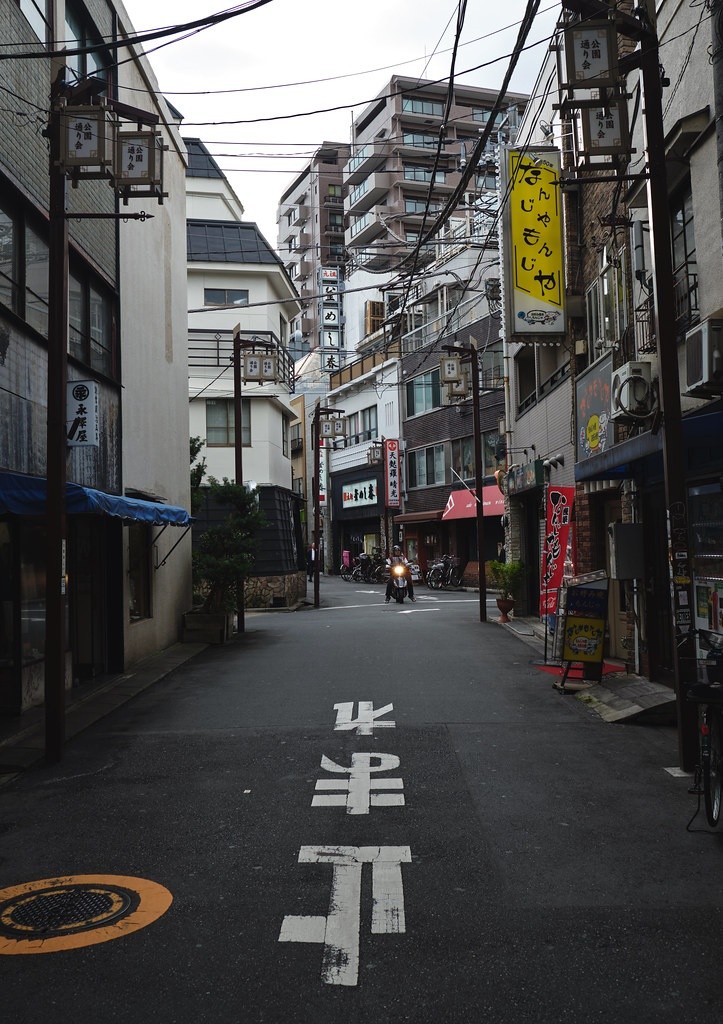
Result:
[391,545,402,551]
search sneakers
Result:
[408,594,417,602]
[384,595,391,604]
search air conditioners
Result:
[685,318,723,396]
[610,360,651,419]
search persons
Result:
[384,545,417,604]
[306,542,317,582]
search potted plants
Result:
[488,560,521,624]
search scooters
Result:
[385,561,414,604]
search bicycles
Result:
[340,552,392,584]
[668,627,723,838]
[425,556,452,589]
[429,554,463,589]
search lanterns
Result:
[547,10,627,119]
[53,97,122,189]
[115,123,169,206]
[439,355,469,402]
[319,415,349,441]
[551,92,636,175]
[242,348,279,387]
[366,446,384,468]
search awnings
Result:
[574,411,723,483]
[0,471,191,531]
